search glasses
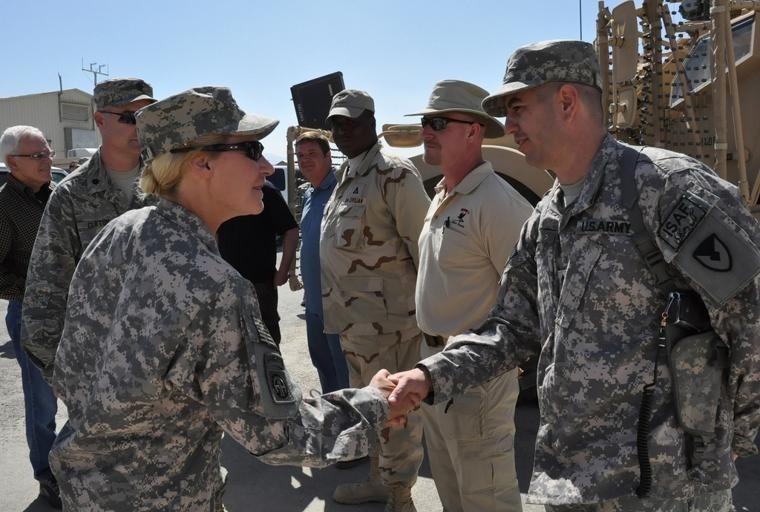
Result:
[419,115,485,133]
[6,149,56,161]
[96,109,137,125]
[168,141,264,163]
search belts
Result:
[424,331,451,348]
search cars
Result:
[267,165,304,247]
[0,160,70,192]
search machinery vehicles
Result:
[284,2,759,405]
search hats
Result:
[94,79,158,108]
[325,89,375,123]
[133,86,279,166]
[479,38,604,118]
[403,78,506,139]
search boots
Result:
[332,470,390,504]
[383,483,417,512]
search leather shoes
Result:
[336,456,368,469]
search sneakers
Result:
[37,472,63,508]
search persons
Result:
[387,38,759,512]
[2,124,63,512]
[19,80,157,386]
[218,180,300,349]
[295,131,371,469]
[322,89,432,512]
[49,86,415,512]
